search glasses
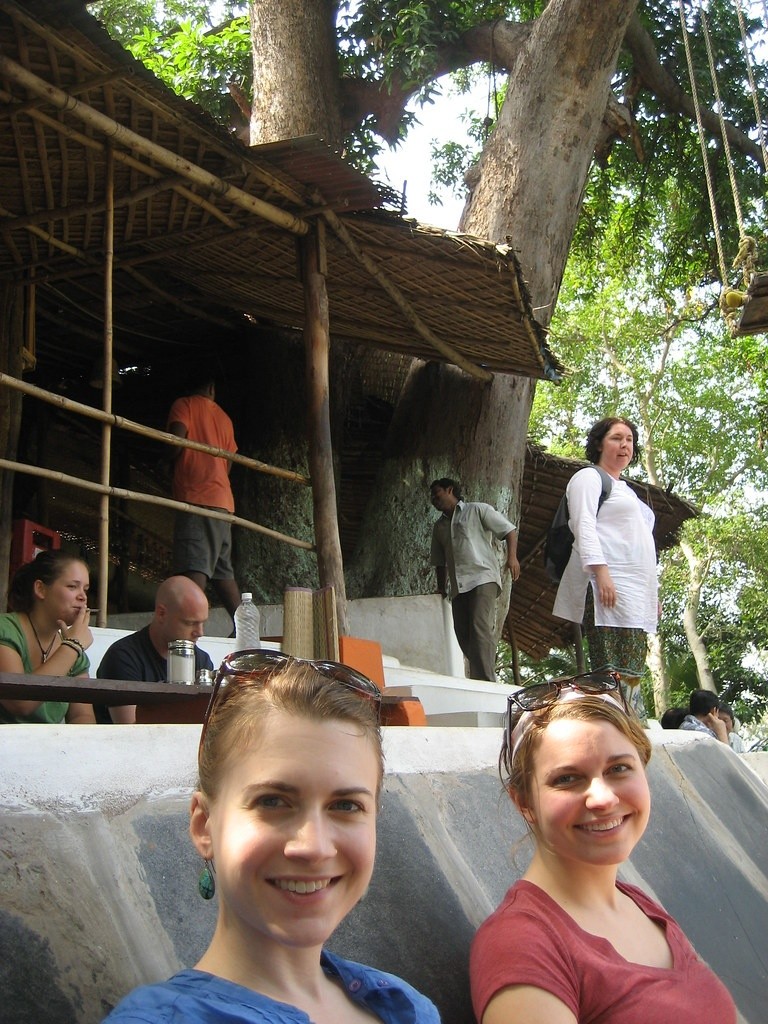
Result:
[506,667,628,761]
[199,649,383,763]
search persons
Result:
[92,575,214,724]
[660,690,746,753]
[159,369,242,639]
[429,478,521,682]
[101,659,443,1024]
[552,418,662,729]
[469,676,751,1024]
[0,549,99,724]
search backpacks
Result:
[545,465,612,567]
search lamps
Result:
[88,349,123,391]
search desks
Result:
[0,671,430,727]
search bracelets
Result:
[61,638,84,657]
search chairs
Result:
[337,635,429,726]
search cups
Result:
[195,668,213,686]
[213,670,227,687]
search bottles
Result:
[234,593,261,651]
[167,639,196,685]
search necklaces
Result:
[25,609,56,663]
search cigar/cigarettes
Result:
[86,608,102,612]
